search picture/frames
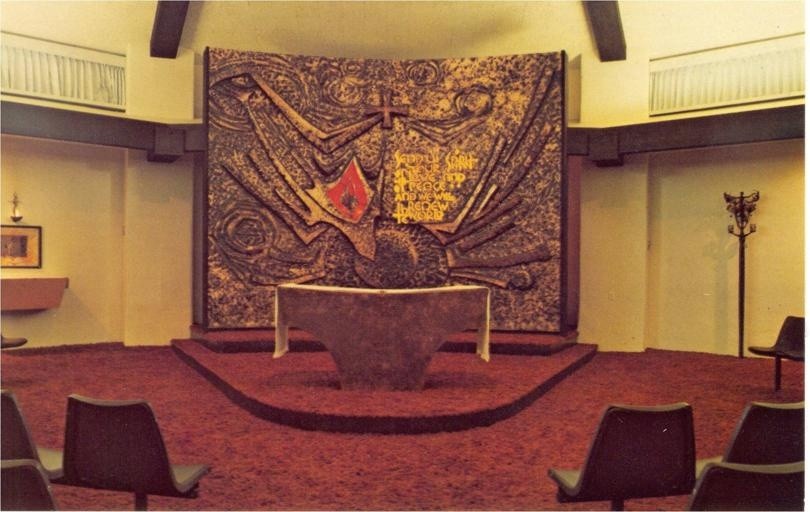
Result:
[0,223,41,270]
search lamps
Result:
[721,188,762,359]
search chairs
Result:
[543,395,804,511]
[1,386,210,508]
[748,313,804,392]
